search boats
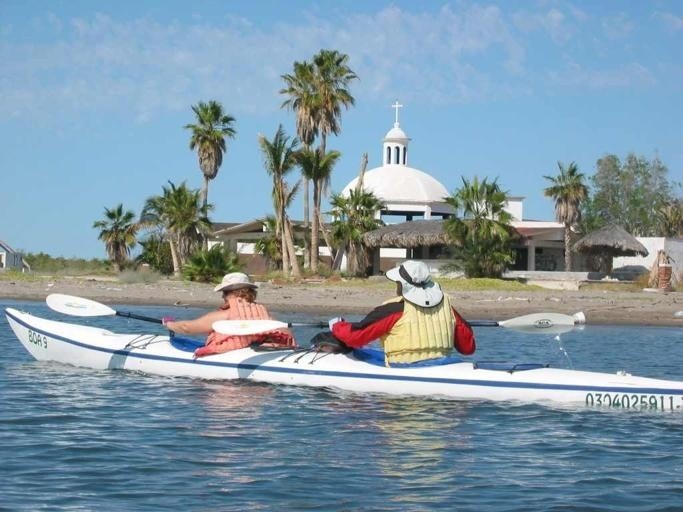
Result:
[2,302,682,420]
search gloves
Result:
[328,315,344,332]
[161,315,176,325]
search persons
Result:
[161,272,298,357]
[328,261,477,368]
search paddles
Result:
[212,313,575,334]
[46,293,162,324]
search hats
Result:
[384,259,445,309]
[213,272,259,292]
